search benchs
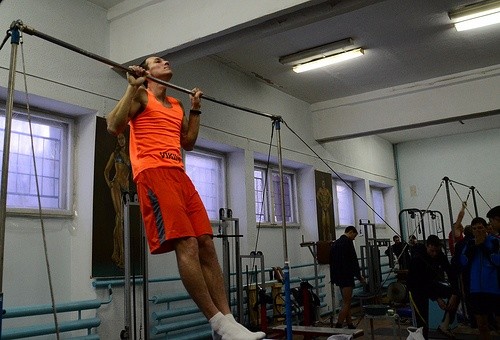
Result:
[272,325,364,340]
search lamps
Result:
[278,38,364,74]
[448,0,500,33]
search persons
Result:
[106,57,266,340]
[385,205,500,340]
[104,132,130,268]
[316,179,333,241]
[330,226,366,329]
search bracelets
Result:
[190,110,202,114]
